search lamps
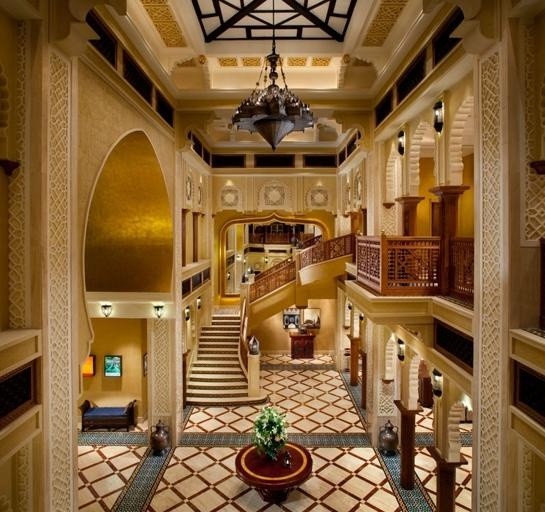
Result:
[232,0,314,151]
[398,338,443,397]
[264,256,268,263]
[154,306,164,318]
[185,296,202,321]
[101,305,113,317]
[398,132,404,156]
[227,271,231,280]
[248,336,259,355]
[433,101,443,133]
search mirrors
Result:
[213,209,334,305]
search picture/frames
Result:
[282,308,321,329]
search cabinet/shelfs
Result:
[289,332,316,359]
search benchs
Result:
[78,400,138,431]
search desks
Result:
[235,440,313,502]
